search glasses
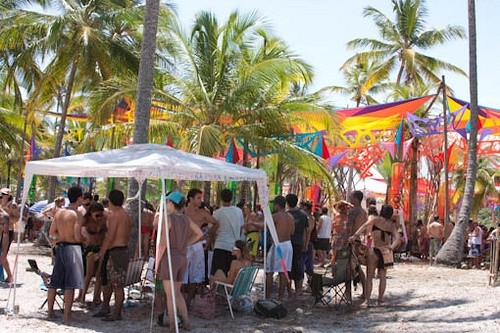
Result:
[94,214,103,217]
[232,246,241,251]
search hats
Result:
[269,196,286,205]
[243,202,252,208]
[0,188,10,194]
[166,192,184,204]
[256,204,261,209]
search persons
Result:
[0,187,30,284]
[403,216,500,270]
[29,184,401,333]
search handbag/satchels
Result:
[232,298,254,312]
[353,265,362,285]
[192,284,216,319]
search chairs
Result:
[307,247,352,311]
[213,266,260,320]
[123,256,155,304]
[28,259,65,311]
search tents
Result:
[5,142,289,333]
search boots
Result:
[156,313,164,326]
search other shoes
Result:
[92,310,111,317]
[100,314,122,321]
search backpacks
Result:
[254,299,287,319]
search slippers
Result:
[3,277,14,283]
